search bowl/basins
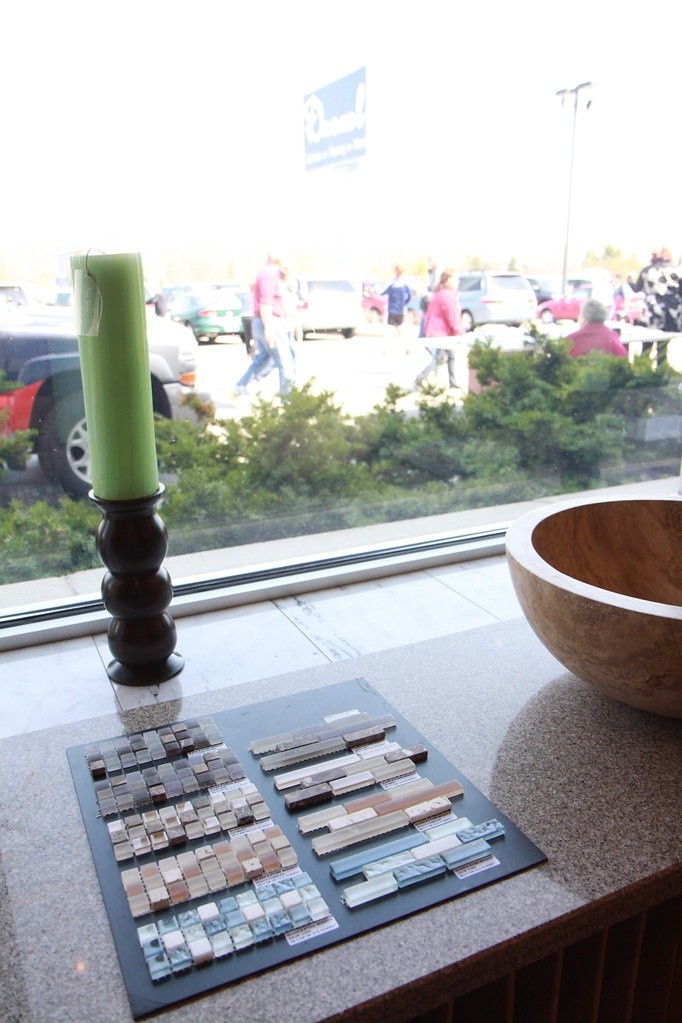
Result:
[504,493,682,720]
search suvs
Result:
[289,277,361,338]
[0,302,214,502]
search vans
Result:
[405,270,537,331]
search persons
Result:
[626,244,682,366]
[378,264,464,393]
[234,252,309,405]
[565,300,628,358]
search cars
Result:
[539,277,650,325]
[160,289,251,344]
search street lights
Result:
[555,81,591,296]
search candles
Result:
[67,250,158,499]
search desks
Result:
[412,327,682,366]
